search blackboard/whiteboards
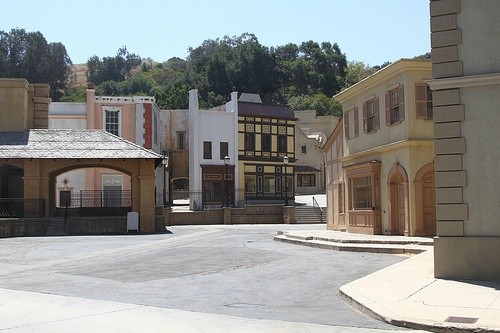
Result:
[126,212,139,231]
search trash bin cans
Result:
[156,215,166,232]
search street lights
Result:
[221,153,232,207]
[160,150,170,208]
[283,154,294,206]
